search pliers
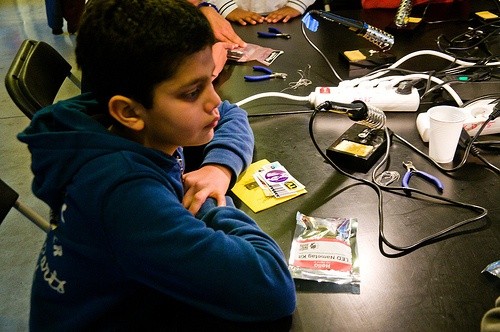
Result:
[402,160,444,194]
[244,66,288,81]
[257,27,291,39]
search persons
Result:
[45,0,452,47]
[15,0,298,332]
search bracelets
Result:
[195,2,219,12]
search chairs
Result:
[1,179,49,234]
[5,39,81,122]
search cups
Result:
[427,106,466,163]
